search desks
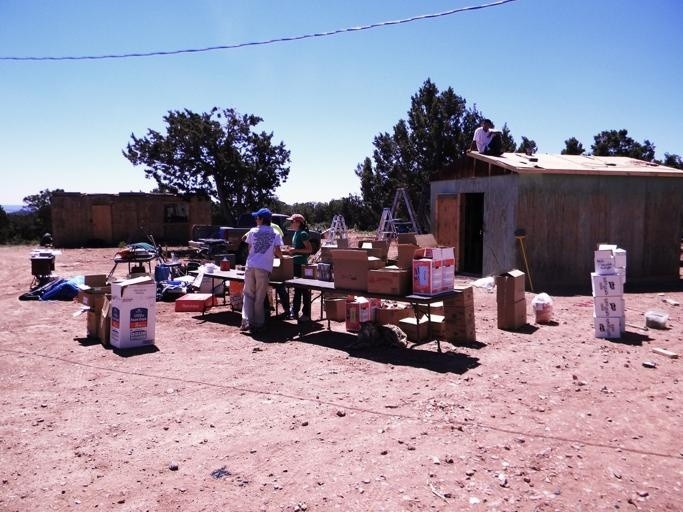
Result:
[188,269,463,356]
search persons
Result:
[465,118,504,157]
[237,208,286,341]
[283,212,313,324]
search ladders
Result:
[384,187,423,238]
[328,213,350,244]
[376,206,399,241]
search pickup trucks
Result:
[191,212,323,266]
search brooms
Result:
[513,226,535,294]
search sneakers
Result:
[280,309,310,321]
[240,320,264,331]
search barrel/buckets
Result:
[155,263,170,281]
[229,281,244,311]
[214,253,236,269]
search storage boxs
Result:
[77,274,157,350]
[591,244,626,339]
[442,268,526,347]
[322,234,455,296]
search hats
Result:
[252,208,272,219]
[286,214,305,222]
